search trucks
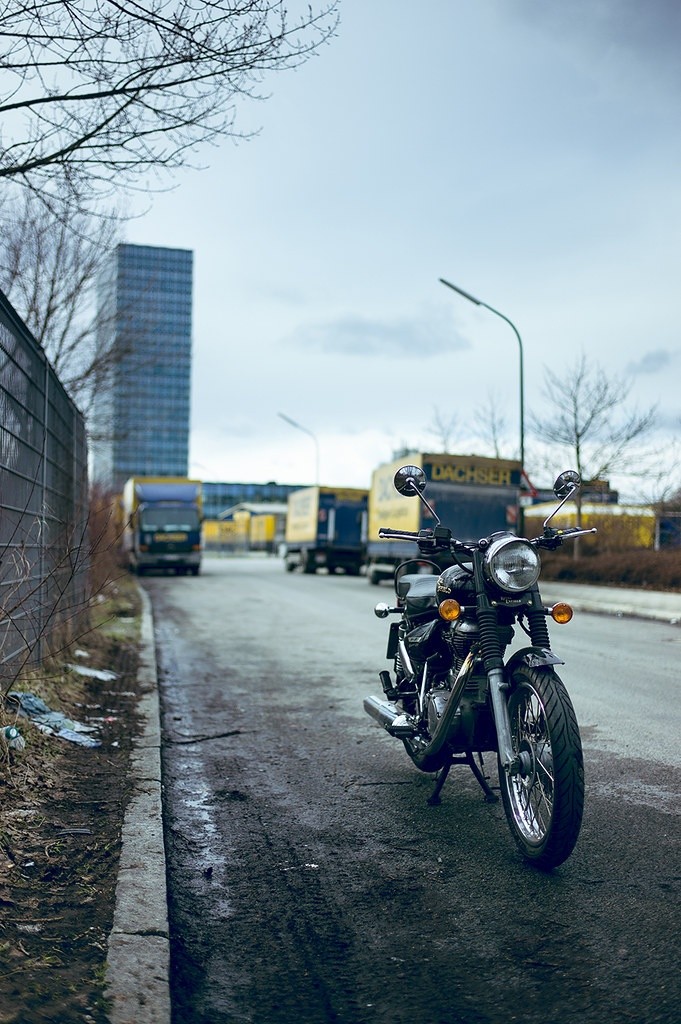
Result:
[280,488,369,579]
[367,451,520,588]
[123,476,206,575]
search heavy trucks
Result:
[522,499,680,558]
[109,493,123,565]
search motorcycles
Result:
[362,464,599,871]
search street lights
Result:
[437,278,525,480]
[276,413,320,486]
